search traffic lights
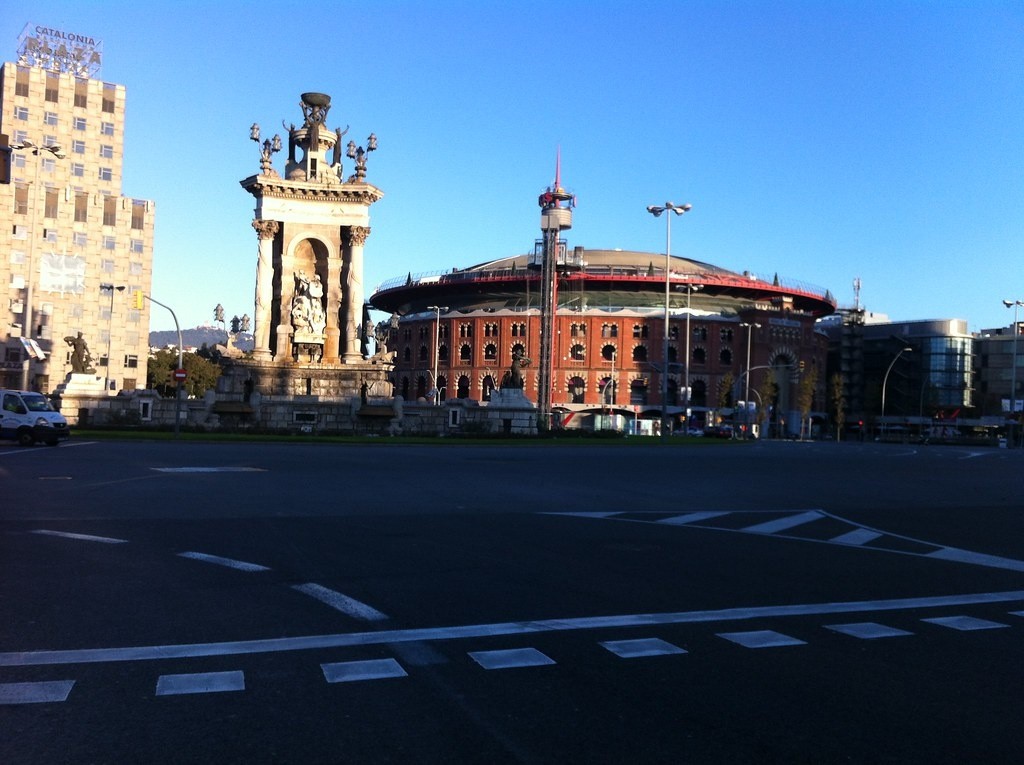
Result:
[133,290,144,310]
[858,418,865,432]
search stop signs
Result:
[174,368,188,382]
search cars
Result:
[0,389,69,447]
[721,426,739,438]
[701,426,732,438]
[670,426,706,438]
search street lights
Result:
[739,322,761,439]
[100,285,127,393]
[880,348,912,441]
[1002,298,1024,448]
[676,284,704,438]
[646,201,691,446]
[9,141,66,392]
[427,305,448,405]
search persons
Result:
[6,397,39,410]
[64,331,95,372]
[511,349,530,387]
